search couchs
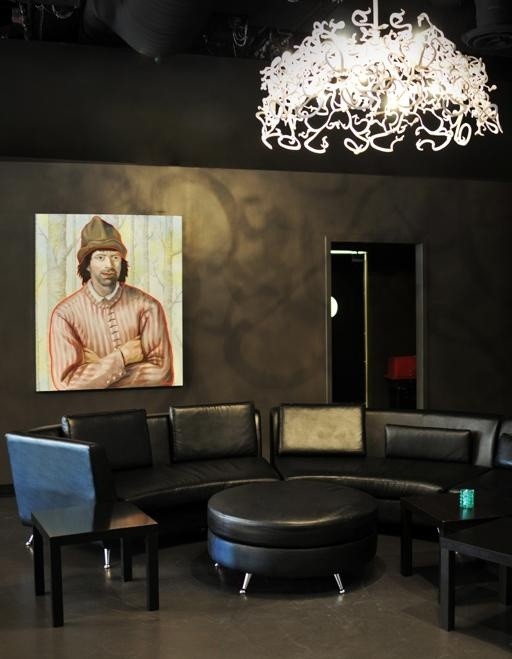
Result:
[4,409,282,570]
[270,405,512,540]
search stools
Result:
[32,501,160,628]
[399,492,503,577]
[206,480,378,595]
[439,515,512,632]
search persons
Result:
[48,215,174,393]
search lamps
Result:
[255,0,503,156]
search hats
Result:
[77,216,127,262]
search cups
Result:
[460,487,475,511]
